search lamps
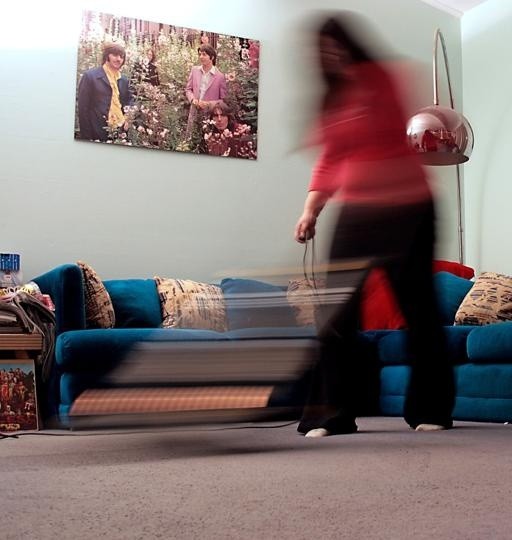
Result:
[407,29,475,265]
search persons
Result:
[0,367,35,431]
[291,12,457,435]
[77,44,133,142]
[132,49,160,101]
[186,46,225,149]
[196,102,252,156]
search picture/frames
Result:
[0,359,42,430]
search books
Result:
[0,309,24,334]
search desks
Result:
[0,333,43,359]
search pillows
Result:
[78,261,324,329]
[432,258,512,325]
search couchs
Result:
[33,264,512,427]
